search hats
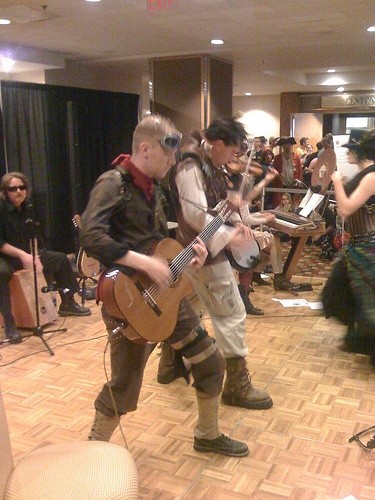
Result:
[341,130,375,150]
[273,136,296,146]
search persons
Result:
[220,136,337,315]
[0,173,92,345]
[157,114,273,410]
[320,130,375,367]
[79,115,250,457]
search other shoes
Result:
[59,302,91,316]
[273,274,301,292]
[5,322,22,344]
[252,271,272,285]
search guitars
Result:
[72,211,102,279]
[94,199,235,345]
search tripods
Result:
[0,228,67,355]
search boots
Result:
[157,343,190,384]
[238,283,264,316]
[221,357,273,410]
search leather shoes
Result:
[193,432,249,457]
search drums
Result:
[228,228,261,272]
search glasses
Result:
[6,185,26,192]
[161,133,184,150]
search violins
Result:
[223,152,278,174]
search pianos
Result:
[264,209,329,285]
